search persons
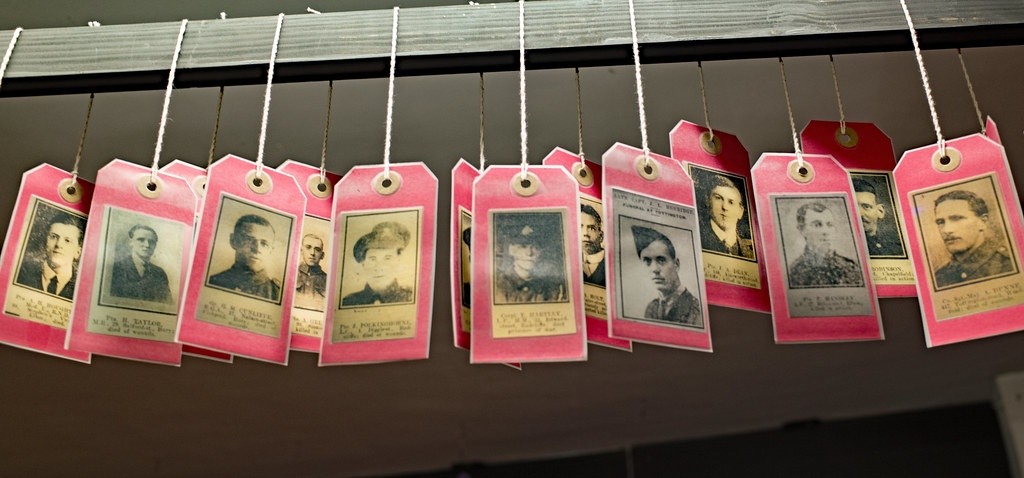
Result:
[11,161,1020,330]
